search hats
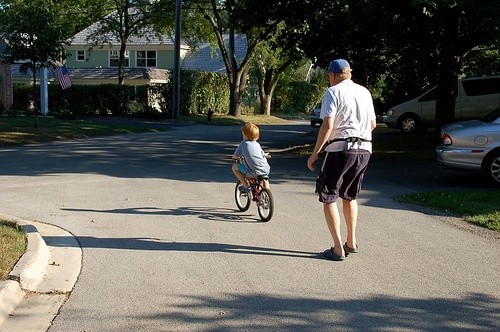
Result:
[327,59,352,73]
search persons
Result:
[307,59,376,261]
[232,123,270,210]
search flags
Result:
[54,64,72,90]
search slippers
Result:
[324,247,345,261]
[343,242,359,253]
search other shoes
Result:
[238,185,253,193]
[262,207,268,209]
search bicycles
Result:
[232,158,278,220]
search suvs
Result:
[384,76,500,134]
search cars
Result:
[311,100,325,127]
[435,116,500,175]
